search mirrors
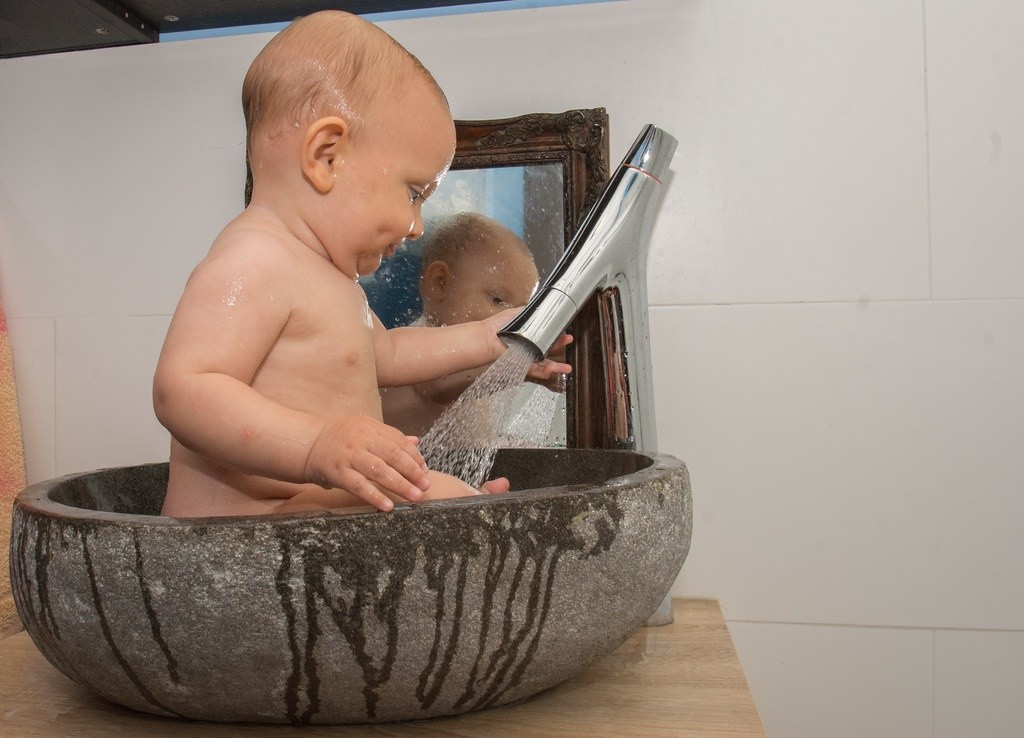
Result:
[245,106,637,451]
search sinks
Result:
[7,445,695,728]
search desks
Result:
[0,599,764,738]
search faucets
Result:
[494,120,681,461]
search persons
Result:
[151,6,579,520]
[380,214,567,451]
